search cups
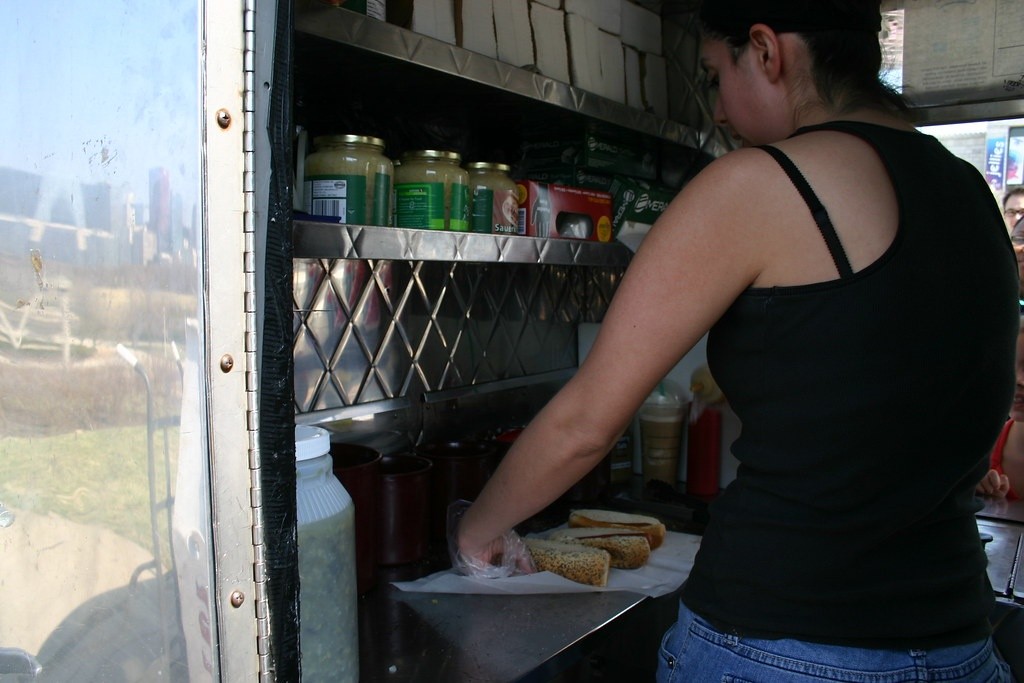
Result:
[416,439,497,553]
[639,411,684,486]
[331,443,382,596]
[383,456,433,564]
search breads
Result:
[523,538,610,587]
[547,527,650,569]
[570,511,665,549]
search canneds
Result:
[467,162,519,234]
[304,135,394,225]
[394,148,470,232]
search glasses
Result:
[1004,208,1024,217]
[1011,235,1024,244]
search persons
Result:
[455,0,1022,682]
[974,187,1024,501]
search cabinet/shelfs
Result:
[288,0,740,268]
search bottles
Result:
[295,425,359,683]
[689,367,724,404]
[603,426,633,485]
